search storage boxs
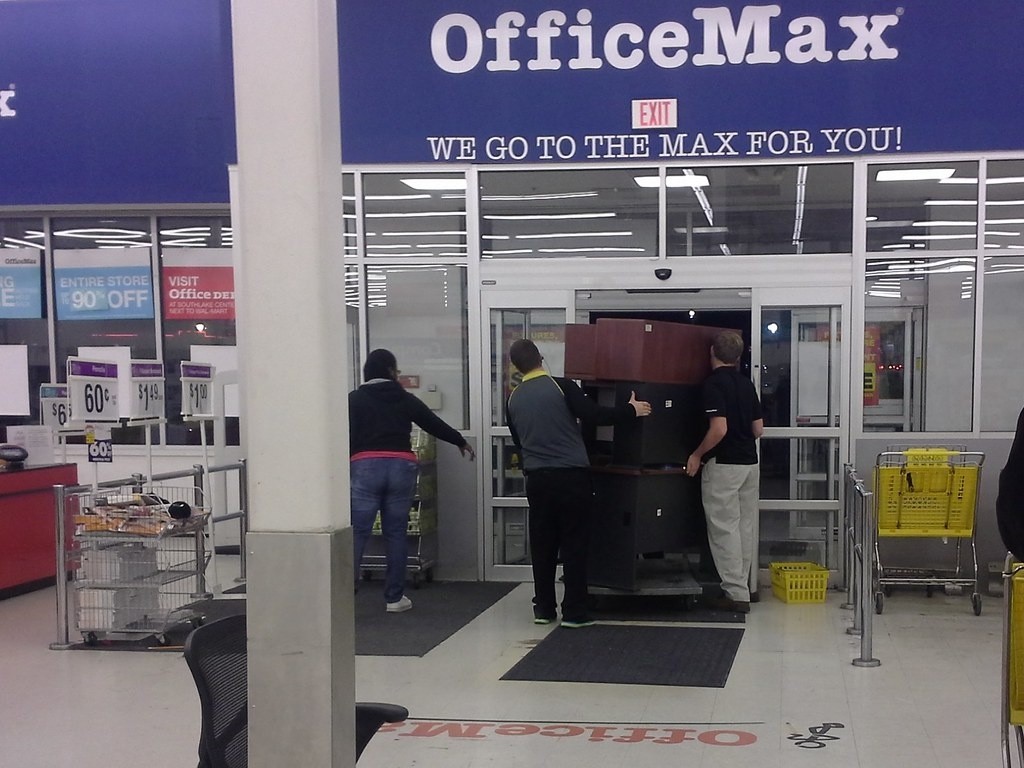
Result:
[768,562,832,598]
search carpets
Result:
[68,580,522,659]
[498,625,746,688]
[577,592,746,624]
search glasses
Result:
[396,367,401,374]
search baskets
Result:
[769,562,829,604]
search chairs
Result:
[185,614,408,768]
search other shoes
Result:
[704,593,760,613]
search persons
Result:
[348,349,476,612]
[995,406,1024,563]
[506,340,652,628]
[687,331,763,612]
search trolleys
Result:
[872,444,985,617]
[75,486,214,649]
[587,549,703,609]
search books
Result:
[78,548,159,629]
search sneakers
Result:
[387,594,413,611]
[560,600,595,627]
[532,604,554,623]
